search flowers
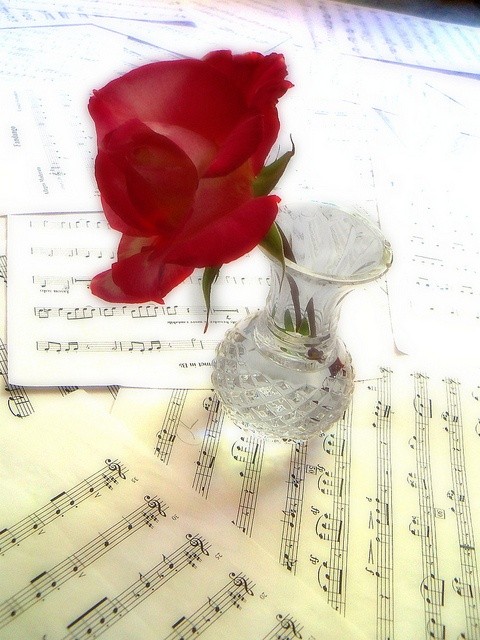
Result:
[88,50,345,378]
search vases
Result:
[212,201,394,445]
